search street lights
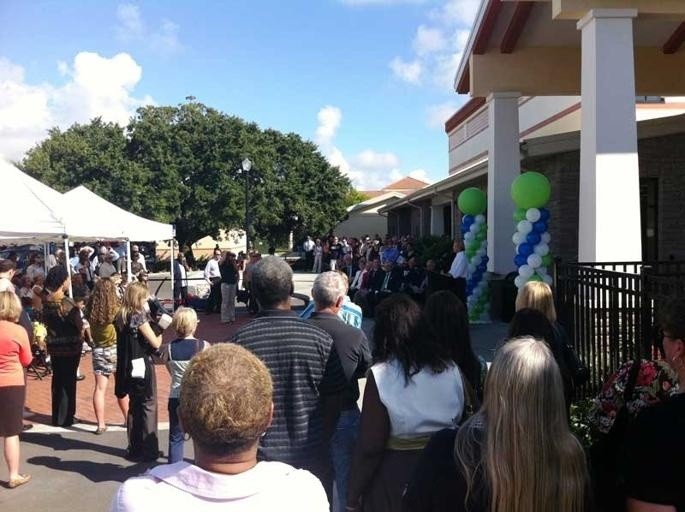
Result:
[234,157,253,254]
[293,210,299,251]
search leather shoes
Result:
[8,473,32,488]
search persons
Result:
[173,253,190,305]
[1,240,150,381]
[41,265,84,426]
[204,248,263,324]
[164,305,212,463]
[113,343,331,511]
[114,280,165,460]
[304,270,373,512]
[354,281,685,512]
[83,277,130,436]
[1,290,32,489]
[303,234,470,300]
[224,254,338,510]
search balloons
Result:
[512,171,553,288]
[457,187,493,323]
